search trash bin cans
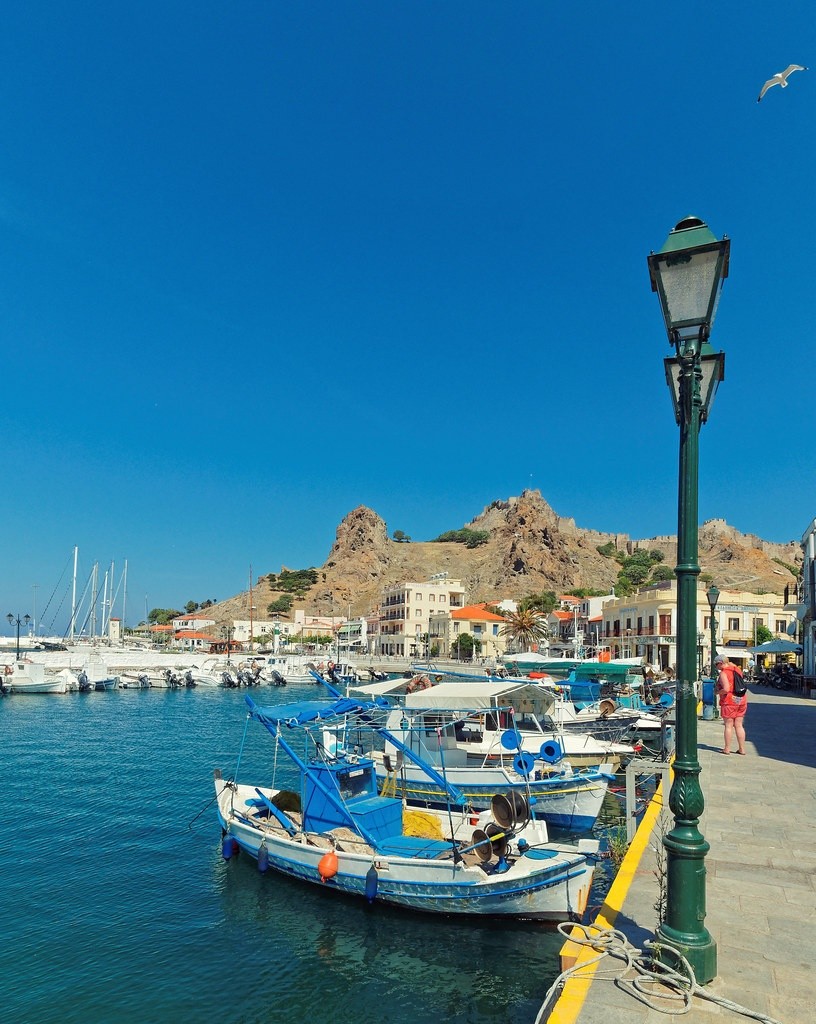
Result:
[702,680,714,719]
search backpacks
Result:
[724,666,747,697]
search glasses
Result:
[715,662,719,666]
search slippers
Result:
[736,750,746,755]
[719,749,730,754]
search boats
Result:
[213,668,602,927]
[0,546,673,833]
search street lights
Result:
[646,215,732,992]
[706,585,720,720]
[589,632,595,658]
[626,628,631,658]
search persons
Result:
[748,658,755,681]
[713,654,747,755]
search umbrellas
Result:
[747,640,804,666]
